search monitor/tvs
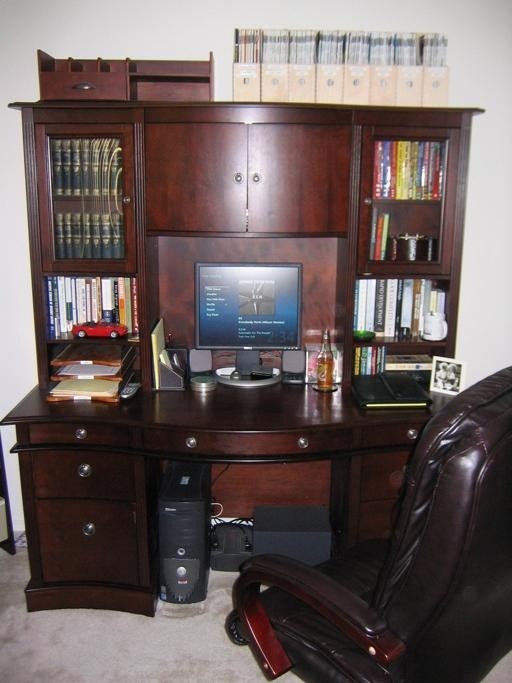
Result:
[194,262,304,388]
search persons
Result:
[445,363,459,390]
[435,362,448,388]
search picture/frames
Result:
[430,354,466,395]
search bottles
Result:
[313,329,338,395]
[316,394,335,425]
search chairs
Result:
[225,367,512,683]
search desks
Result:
[0,376,462,617]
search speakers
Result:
[252,505,333,566]
[190,349,212,377]
[281,349,306,385]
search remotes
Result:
[121,381,141,399]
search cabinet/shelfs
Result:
[334,105,484,384]
[7,100,159,392]
[37,48,215,102]
[144,101,349,239]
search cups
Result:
[422,312,447,342]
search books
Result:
[43,137,139,339]
[234,26,448,69]
[354,138,448,374]
[352,373,433,409]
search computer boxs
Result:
[157,461,211,604]
